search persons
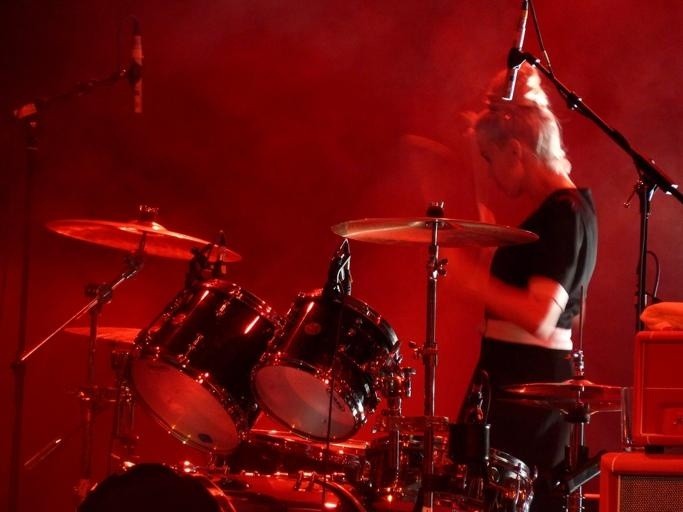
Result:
[416,56,599,511]
[77,462,220,511]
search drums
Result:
[76,463,232,506]
[366,431,444,512]
[127,278,281,456]
[206,429,373,512]
[251,288,403,443]
[421,445,534,512]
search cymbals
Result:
[64,326,143,345]
[44,218,243,262]
[330,215,541,248]
[495,378,626,412]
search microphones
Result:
[499,0,534,101]
[341,237,355,295]
[130,25,147,115]
